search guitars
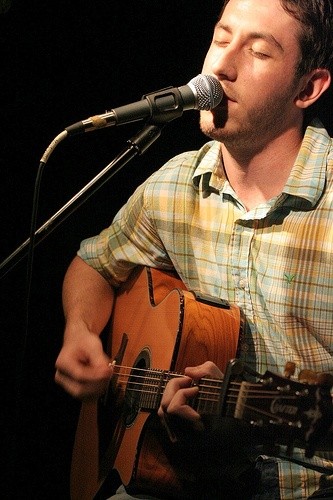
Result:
[66,264,332,500]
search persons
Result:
[52,0,333,499]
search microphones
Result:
[64,75,223,135]
